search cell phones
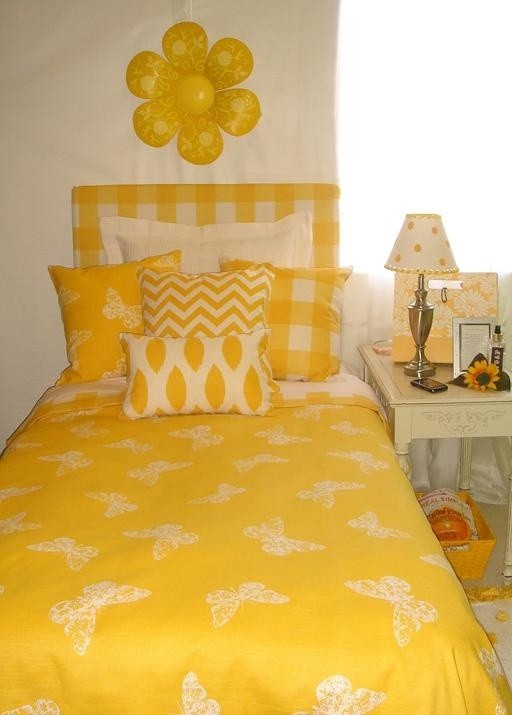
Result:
[410,377,448,393]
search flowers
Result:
[446,352,511,393]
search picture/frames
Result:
[451,316,495,380]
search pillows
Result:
[137,266,281,392]
[115,328,277,419]
[47,248,185,392]
[218,253,354,383]
[97,211,315,273]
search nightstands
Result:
[355,343,512,585]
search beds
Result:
[0,181,512,715]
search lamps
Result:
[380,212,460,376]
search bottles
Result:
[488,325,504,373]
